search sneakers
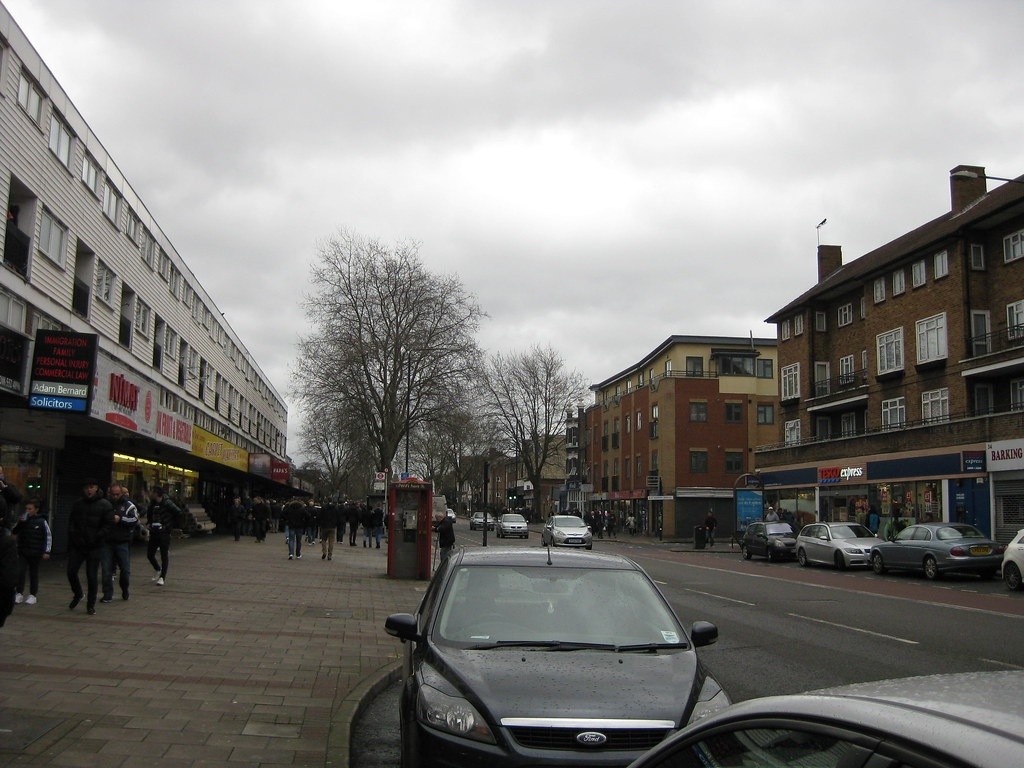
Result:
[14,592,23,603]
[25,594,37,604]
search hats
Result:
[769,507,773,510]
[83,478,98,486]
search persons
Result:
[63,478,146,615]
[549,508,617,539]
[0,464,52,627]
[230,496,384,560]
[860,506,879,533]
[432,512,455,561]
[626,513,636,535]
[502,506,530,520]
[147,485,182,585]
[704,512,718,545]
[765,507,786,520]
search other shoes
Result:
[152,570,161,580]
[297,555,302,559]
[101,595,112,603]
[350,542,357,546]
[122,589,129,600]
[289,553,293,559]
[88,606,96,615]
[328,557,331,560]
[376,543,380,548]
[156,577,164,585]
[322,553,326,559]
[70,594,85,609]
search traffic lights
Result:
[508,489,516,499]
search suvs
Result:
[796,520,886,571]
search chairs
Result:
[458,573,642,619]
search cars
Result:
[432,494,457,527]
[737,520,801,563]
[869,522,1024,590]
[470,512,496,531]
[383,547,1024,768]
[541,515,593,550]
[496,513,529,539]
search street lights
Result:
[405,347,460,477]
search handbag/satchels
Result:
[603,526,606,531]
[626,520,629,526]
